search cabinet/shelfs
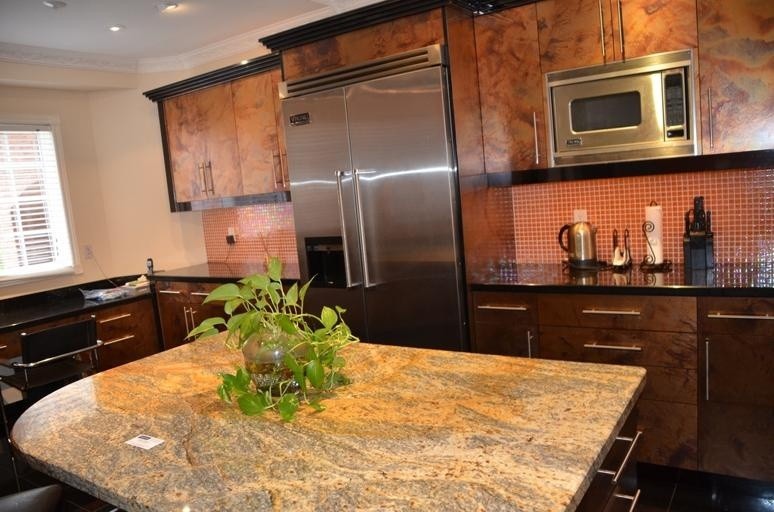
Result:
[535,0,701,75]
[697,0,774,153]
[162,87,244,204]
[474,4,547,174]
[95,294,160,372]
[152,260,300,347]
[467,290,537,357]
[697,295,774,482]
[537,294,697,470]
[230,69,290,196]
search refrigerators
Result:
[281,66,469,351]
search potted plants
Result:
[183,257,361,422]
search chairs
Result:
[11,317,104,396]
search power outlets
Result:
[82,244,93,260]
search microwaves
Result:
[542,48,701,167]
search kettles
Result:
[558,222,607,272]
